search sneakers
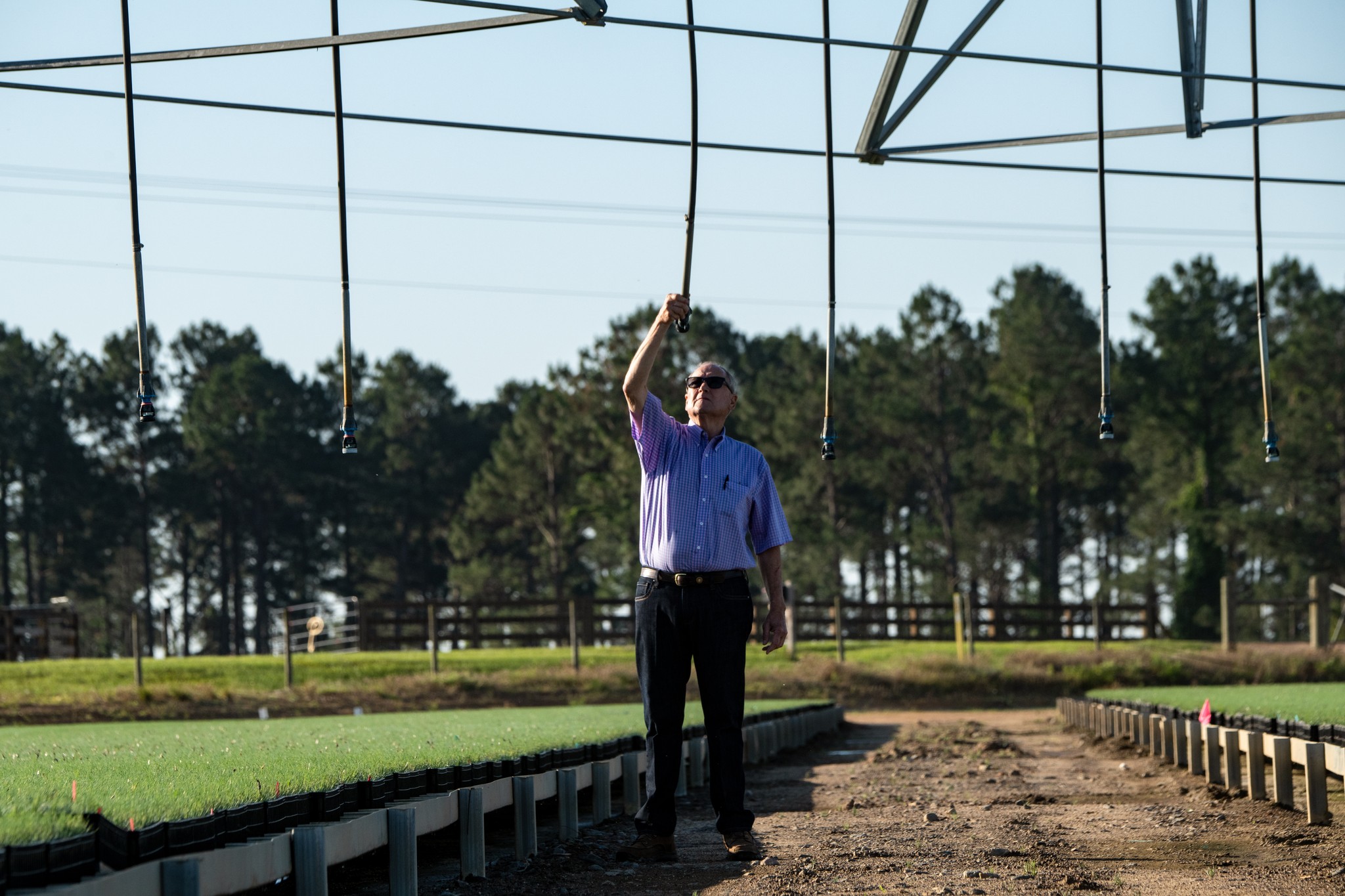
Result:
[616,827,680,863]
[722,830,762,862]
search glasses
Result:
[640,567,745,587]
[685,376,734,395]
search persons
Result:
[622,289,792,868]
